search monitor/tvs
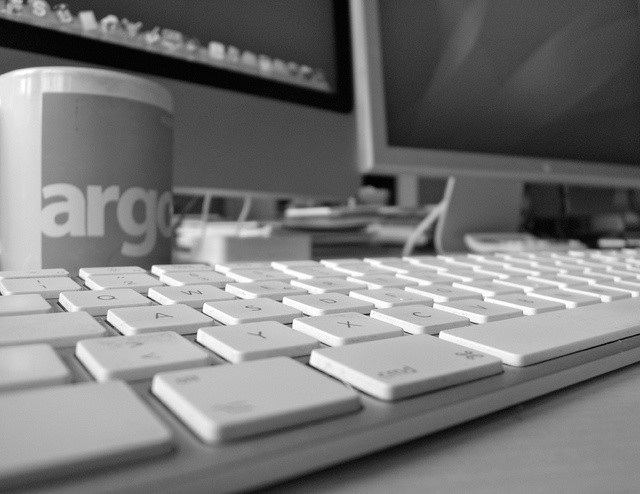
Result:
[1,0,351,118]
[352,0,640,253]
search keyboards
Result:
[0,248,639,489]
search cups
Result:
[0,66,175,278]
